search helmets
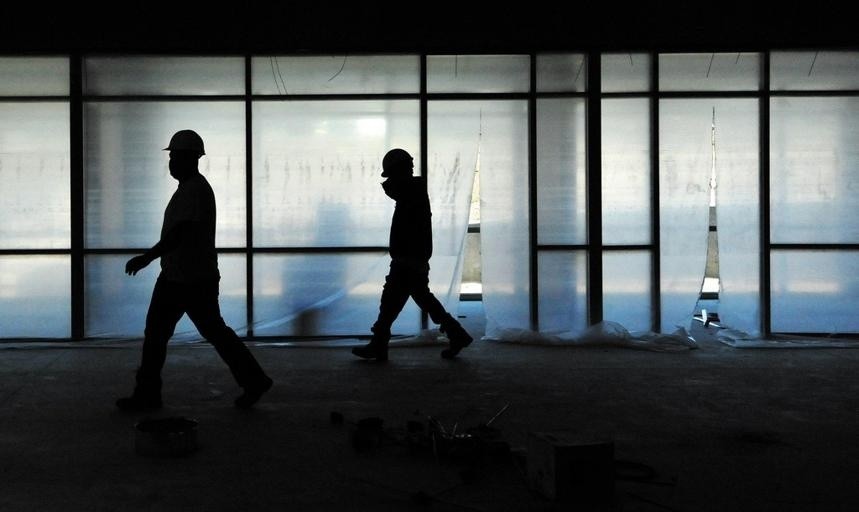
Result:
[381,148,414,177]
[162,130,206,158]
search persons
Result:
[116,128,273,415]
[349,147,473,362]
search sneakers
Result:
[234,372,273,409]
[115,388,163,412]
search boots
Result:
[441,324,473,358]
[352,330,391,362]
